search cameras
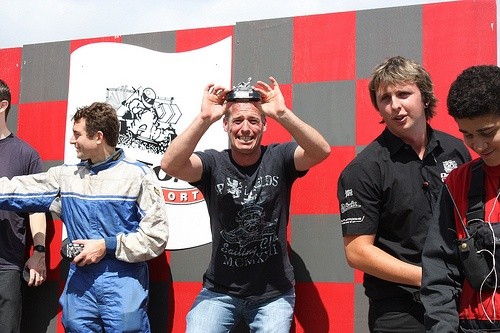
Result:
[60,243,84,261]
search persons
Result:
[0,79,46,333]
[419,64,500,333]
[337,56,472,333]
[160,77,331,333]
[0,102,169,333]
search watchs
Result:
[34,245,46,252]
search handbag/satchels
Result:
[456,157,500,292]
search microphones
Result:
[424,181,431,187]
